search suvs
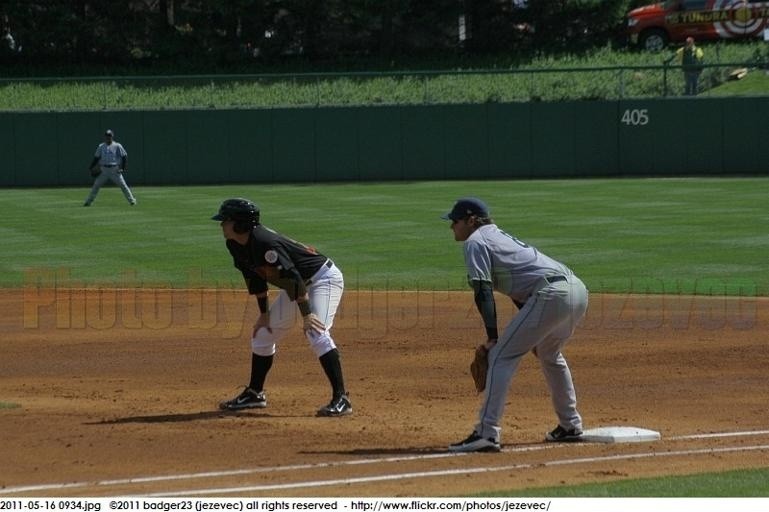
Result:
[626,0,768,52]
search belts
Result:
[104,165,116,168]
[545,275,567,284]
[306,260,332,285]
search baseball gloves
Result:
[470,344,489,393]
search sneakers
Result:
[545,426,584,442]
[317,391,353,417]
[448,431,501,453]
[219,387,267,408]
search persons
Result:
[82,129,139,209]
[212,197,355,417]
[663,38,704,95]
[441,197,590,452]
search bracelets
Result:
[255,296,270,313]
[298,300,312,316]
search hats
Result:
[439,198,489,222]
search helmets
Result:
[209,198,259,232]
[104,129,113,137]
